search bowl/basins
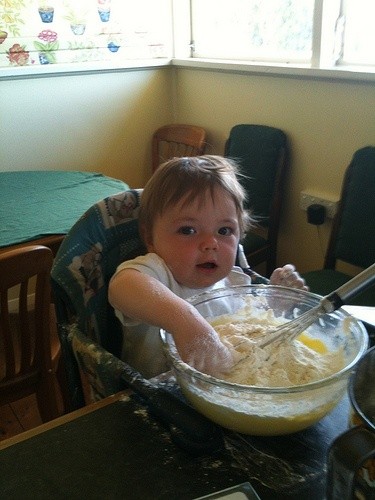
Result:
[160,283,368,438]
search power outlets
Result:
[299,190,342,219]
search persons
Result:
[108,154,309,379]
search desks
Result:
[0,305,375,500]
[0,170,131,258]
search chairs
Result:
[300,146,375,307]
[0,245,58,422]
[151,123,207,173]
[222,124,287,277]
[50,188,270,411]
